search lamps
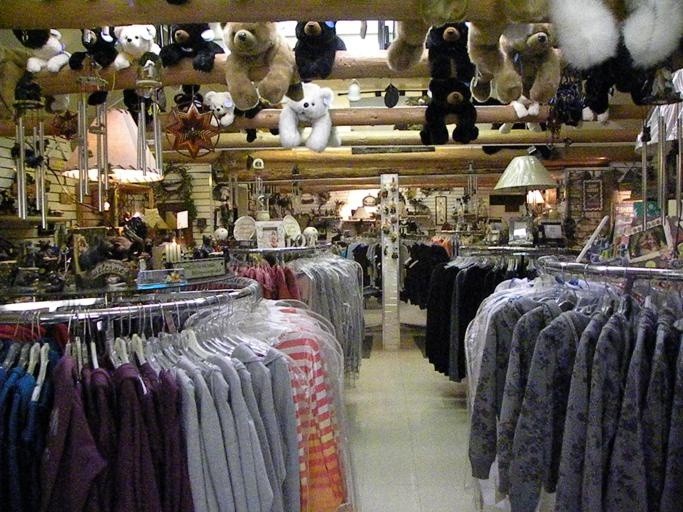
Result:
[493,156,559,193]
[348,78,360,102]
[60,108,164,183]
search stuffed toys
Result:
[13,0,683,153]
[13,1,683,152]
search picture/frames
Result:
[509,217,533,244]
[435,195,447,226]
[541,223,562,240]
[255,221,285,248]
[582,179,604,212]
[622,218,674,263]
